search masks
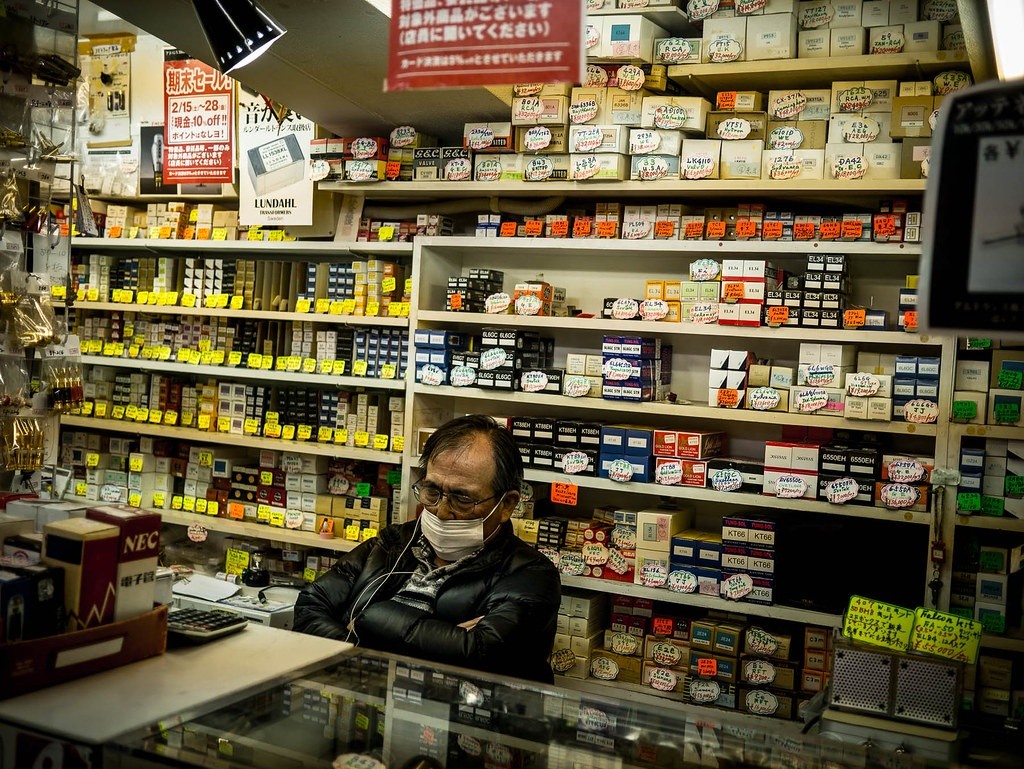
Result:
[420,492,508,562]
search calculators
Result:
[167,607,249,646]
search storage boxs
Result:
[317,0,964,182]
[2,196,1022,719]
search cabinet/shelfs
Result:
[51,240,413,599]
[316,50,970,734]
[937,327,1024,756]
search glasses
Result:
[412,475,501,514]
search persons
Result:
[290,416,562,769]
